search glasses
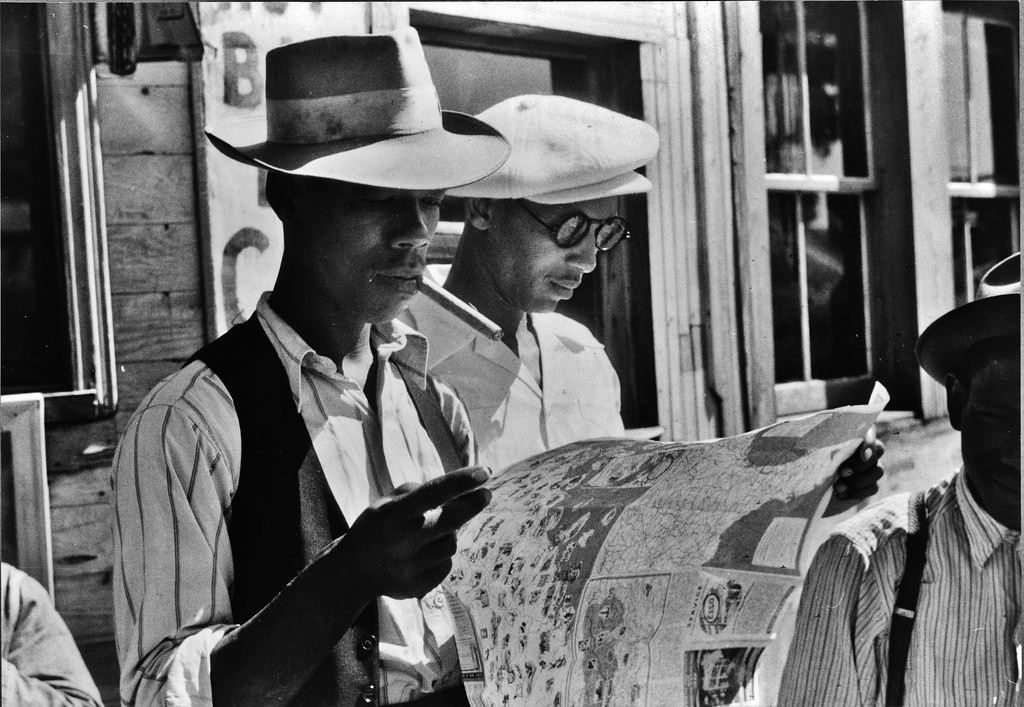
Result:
[516,200,631,252]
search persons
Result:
[107,26,884,707]
[1,560,105,706]
[774,251,1024,707]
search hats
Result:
[914,252,1020,387]
[446,94,659,205]
[204,27,512,190]
